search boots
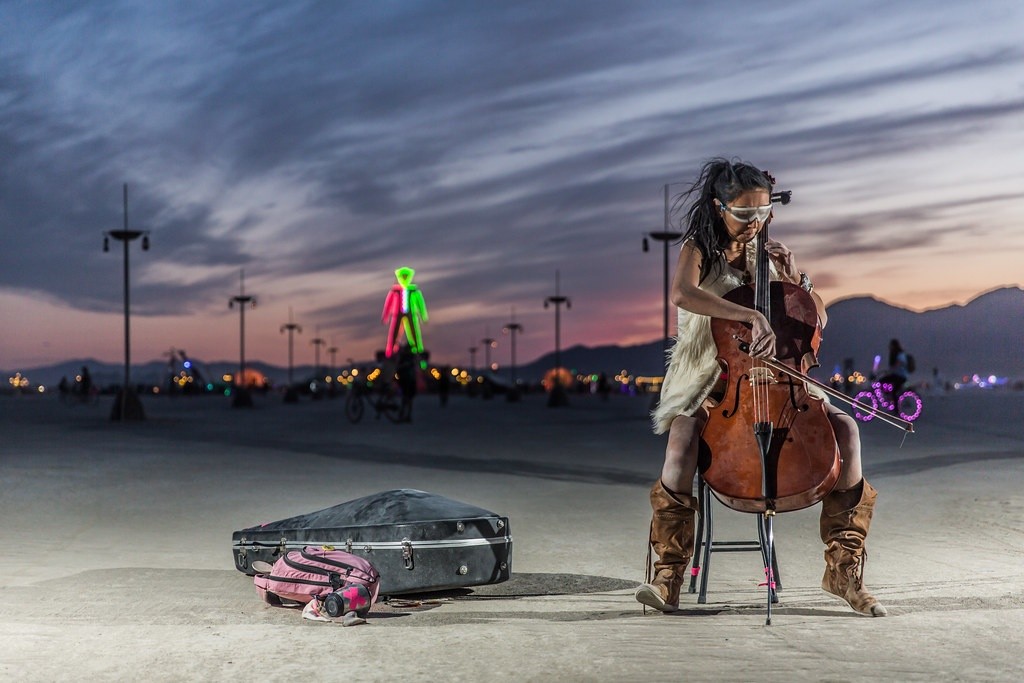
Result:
[820,480,886,618]
[637,481,702,613]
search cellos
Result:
[697,170,843,625]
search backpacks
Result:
[255,548,379,612]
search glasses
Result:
[722,203,771,221]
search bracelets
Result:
[798,272,814,295]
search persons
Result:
[637,157,889,618]
[59,366,102,404]
[828,356,943,395]
[175,366,612,406]
[393,344,418,424]
[871,340,909,415]
